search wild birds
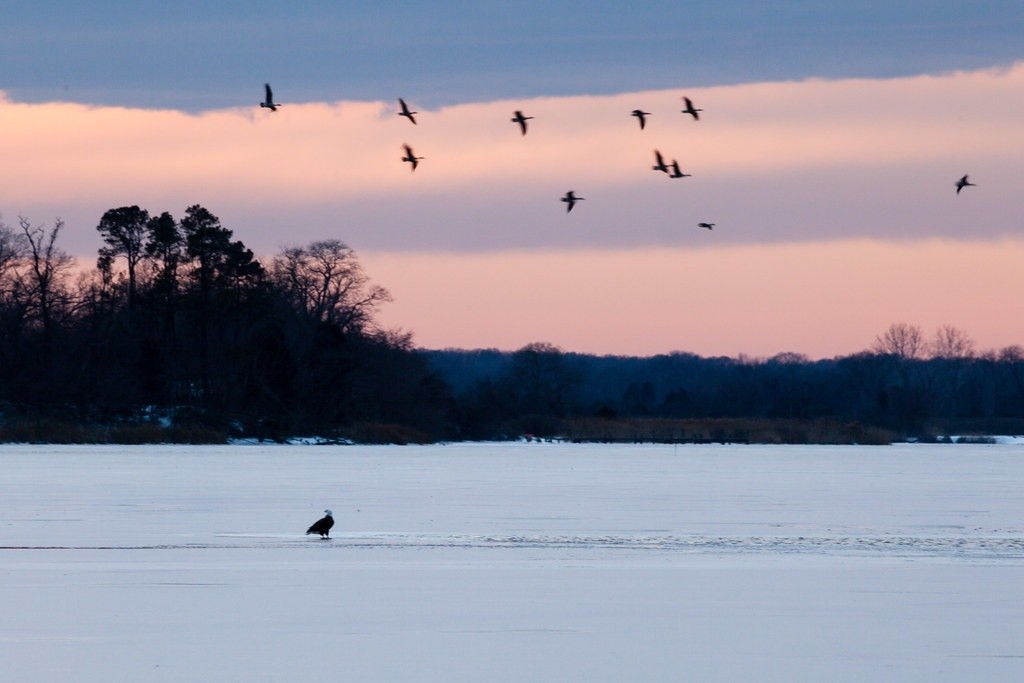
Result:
[669,159,691,178]
[402,143,425,172]
[955,175,976,194]
[305,510,334,539]
[631,110,651,130]
[561,191,585,213]
[698,223,715,230]
[261,83,282,111]
[398,98,418,125]
[511,111,534,137]
[681,96,703,120]
[652,150,676,173]
